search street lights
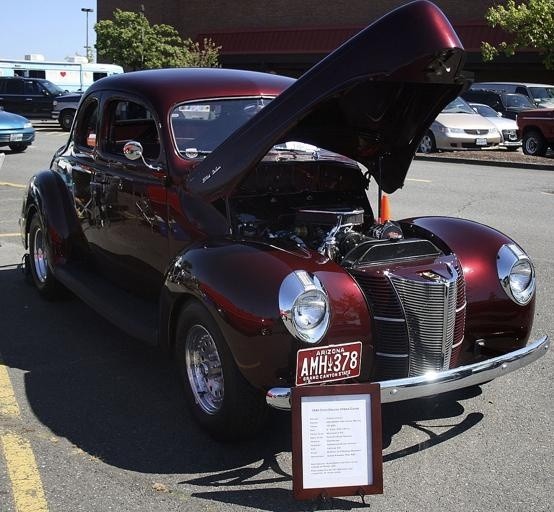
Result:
[80,7,94,60]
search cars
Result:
[419,83,554,156]
[10,0,550,438]
[0,105,35,153]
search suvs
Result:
[51,93,83,129]
[0,76,86,131]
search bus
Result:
[0,60,124,97]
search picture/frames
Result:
[291,383,383,500]
